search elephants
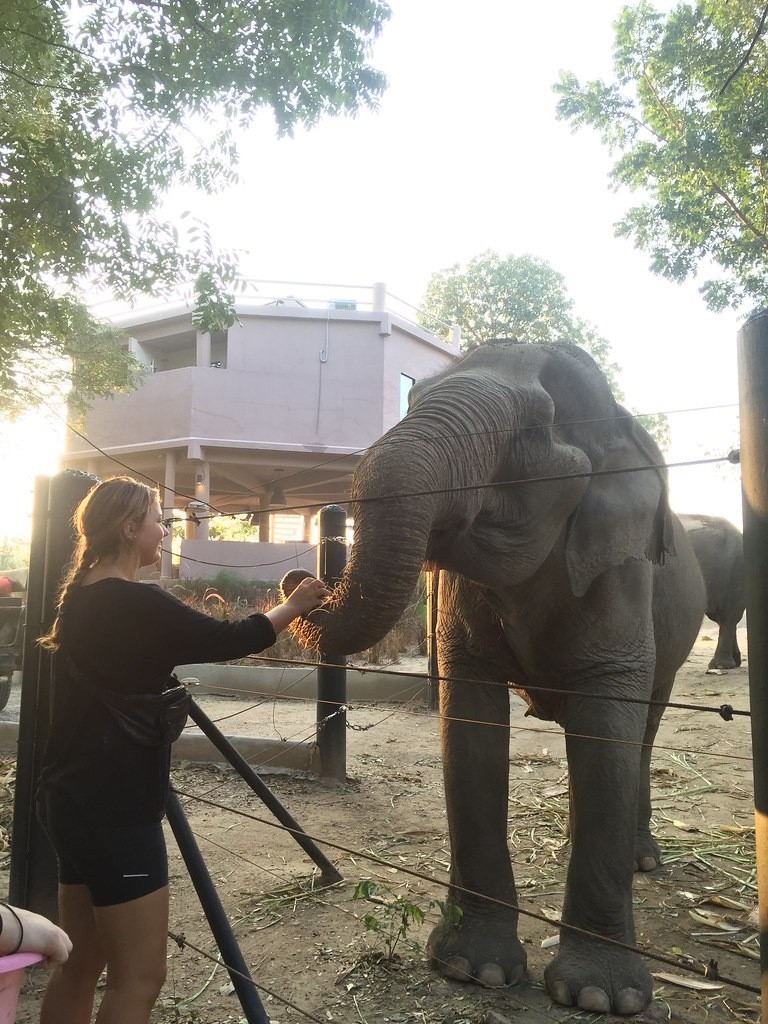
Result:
[674,514,745,671]
[280,338,708,1016]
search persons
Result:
[35,475,332,1023]
[0,903,74,964]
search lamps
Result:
[250,497,260,525]
[270,468,288,507]
[196,473,203,485]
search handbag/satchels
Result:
[95,675,192,747]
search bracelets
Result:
[0,902,24,955]
